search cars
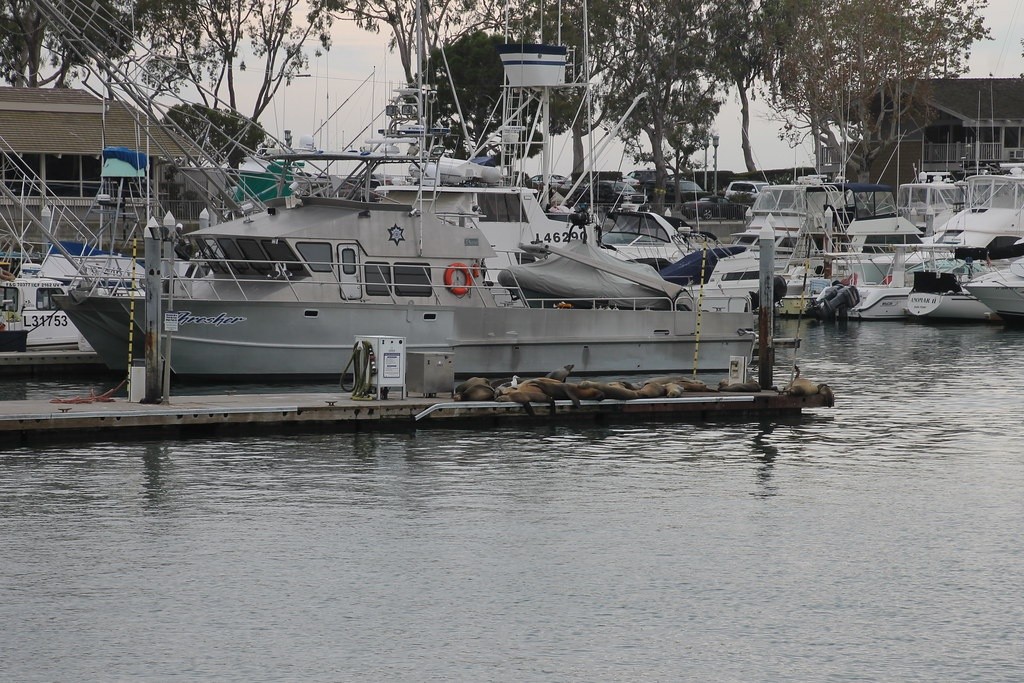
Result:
[525,174,571,197]
[682,194,751,221]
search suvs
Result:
[724,181,769,200]
[642,180,711,203]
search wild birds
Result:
[500,375,519,389]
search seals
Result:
[451,364,834,419]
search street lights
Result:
[712,135,720,196]
[702,136,710,190]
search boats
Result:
[0,0,1024,384]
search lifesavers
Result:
[445,262,472,295]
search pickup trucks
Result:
[569,180,648,205]
